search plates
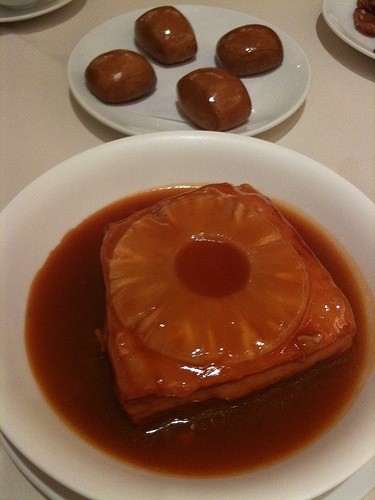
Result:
[0,0,72,21]
[321,0,375,59]
[67,4,311,136]
[0,129,375,500]
